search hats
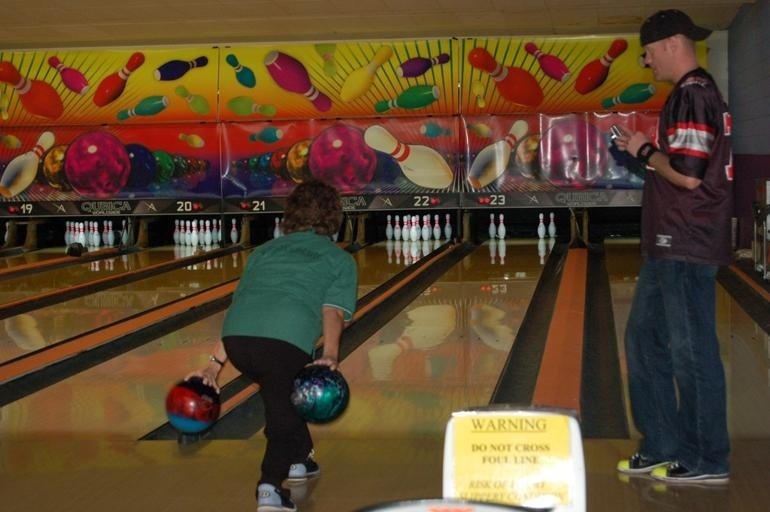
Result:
[640,8,713,48]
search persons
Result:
[183,179,358,511]
[608,8,734,487]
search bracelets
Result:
[637,142,659,164]
[209,354,224,366]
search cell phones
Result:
[610,124,623,138]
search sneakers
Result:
[617,449,682,474]
[256,480,297,512]
[648,461,731,480]
[285,454,321,482]
